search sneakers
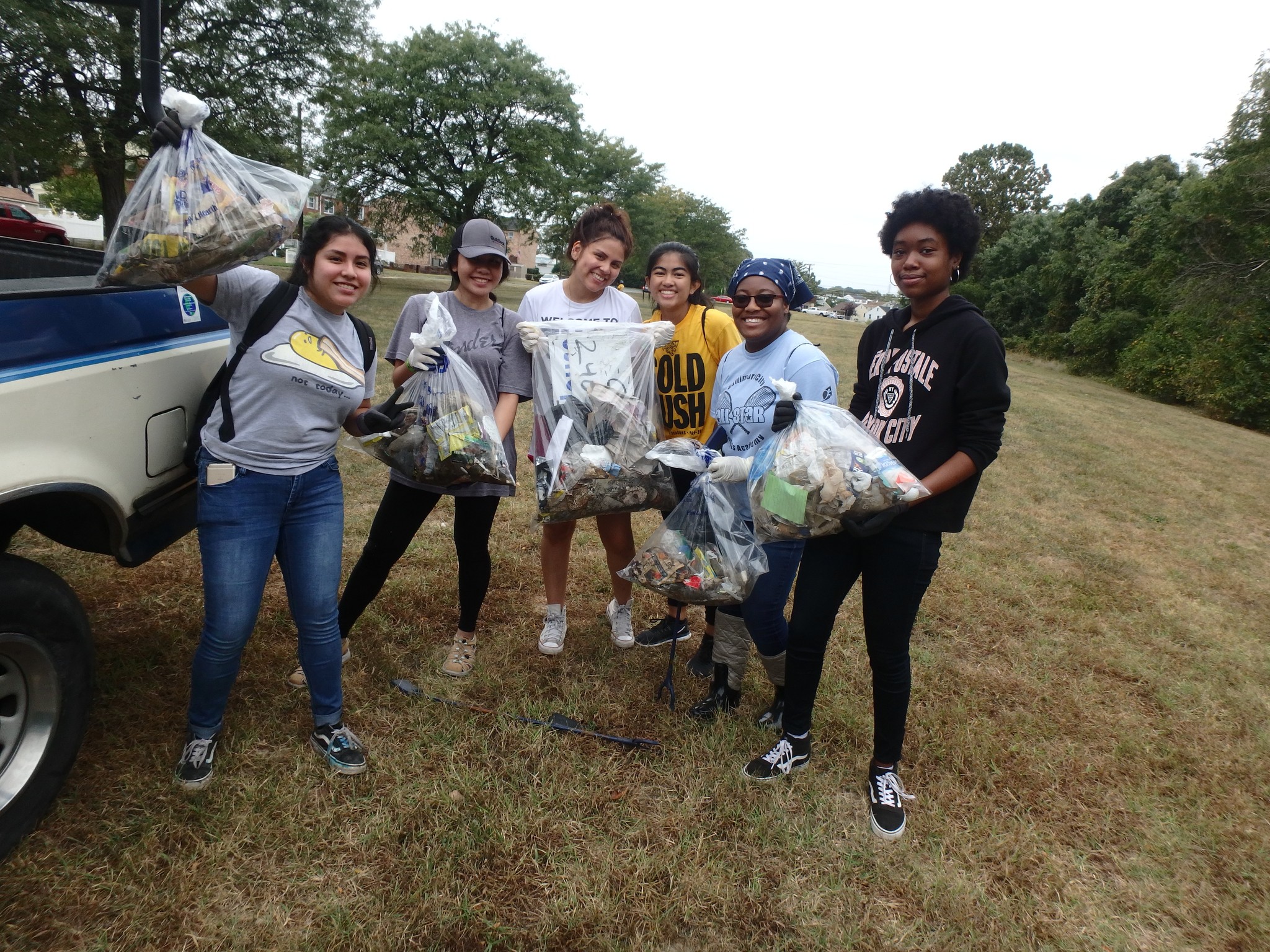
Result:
[741,730,811,784]
[634,613,692,647]
[538,603,567,655]
[868,757,916,841]
[173,733,220,793]
[684,633,715,681]
[309,722,367,775]
[606,595,635,648]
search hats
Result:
[451,218,512,266]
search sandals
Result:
[441,633,477,677]
[287,636,351,689]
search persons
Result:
[285,218,532,689]
[517,202,677,656]
[687,257,840,730]
[633,240,748,679]
[743,186,1013,842]
[146,106,416,789]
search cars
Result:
[827,312,837,319]
[791,306,801,312]
[643,283,651,293]
[711,295,733,304]
[0,202,70,247]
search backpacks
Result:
[183,279,376,470]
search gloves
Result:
[515,320,545,353]
[839,503,911,541]
[408,344,440,371]
[640,321,676,350]
[356,386,415,437]
[771,392,803,433]
[149,109,184,149]
[708,456,755,483]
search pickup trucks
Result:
[802,307,830,317]
[0,232,238,875]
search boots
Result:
[754,645,787,731]
[687,606,752,722]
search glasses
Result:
[731,293,786,308]
